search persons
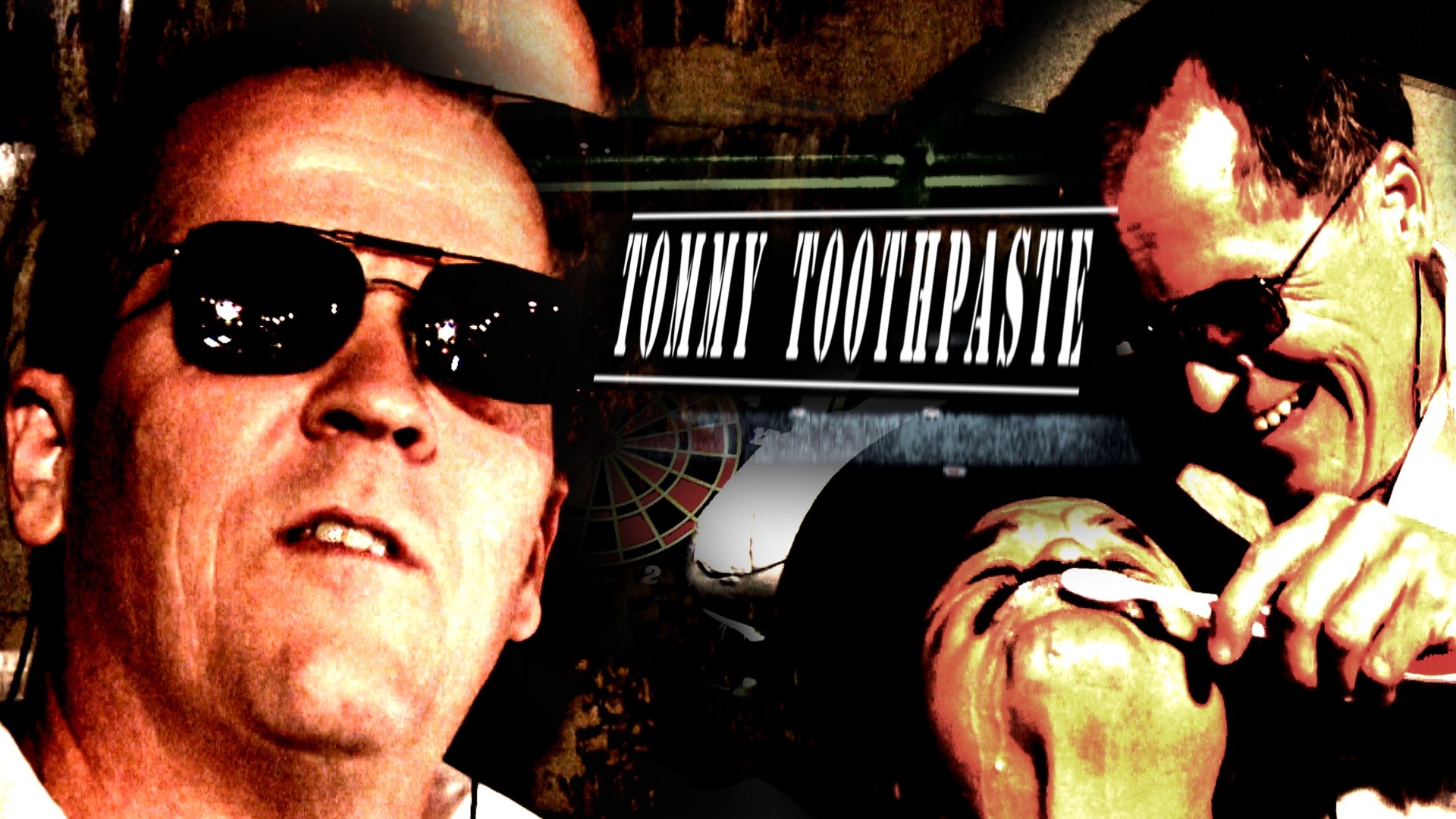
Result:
[1,54,570,819]
[922,493,1226,818]
[1045,3,1454,818]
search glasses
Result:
[32,214,610,405]
[1124,149,1384,368]
